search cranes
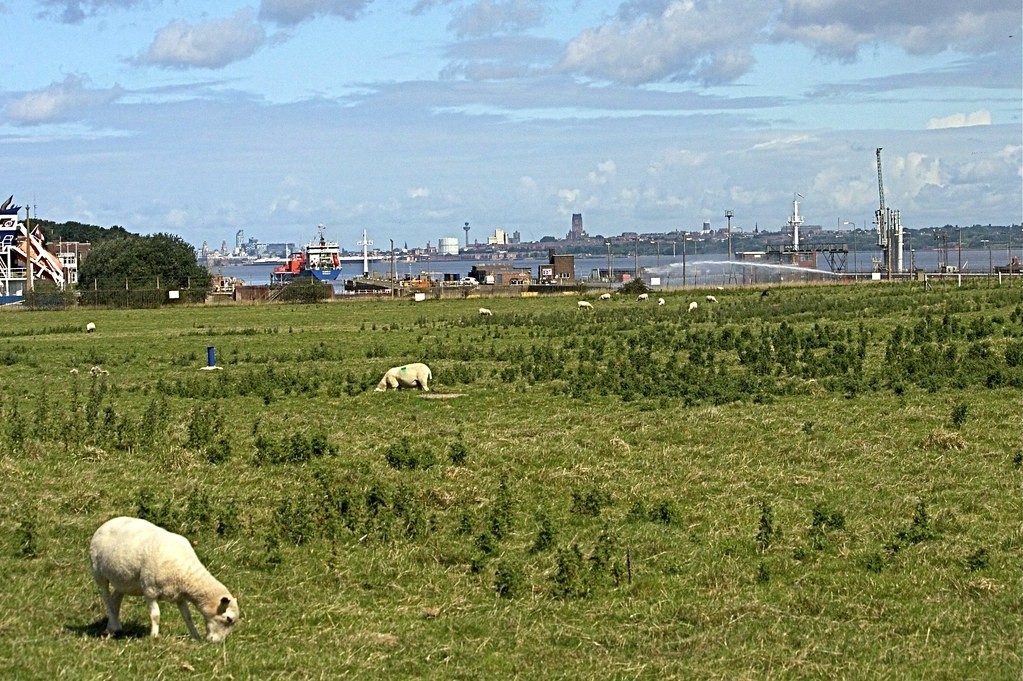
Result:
[874,146,892,282]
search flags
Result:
[35,227,47,243]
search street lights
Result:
[631,235,639,278]
[425,256,432,287]
[902,230,913,280]
[23,203,32,291]
[388,238,394,301]
[604,240,611,277]
[649,239,661,267]
[682,232,693,287]
[954,228,962,275]
[843,220,857,273]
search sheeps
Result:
[658,297,665,306]
[88,516,241,644]
[705,295,719,304]
[478,307,492,317]
[688,302,698,312]
[598,293,611,301]
[373,363,434,392]
[577,301,594,310]
[635,293,649,302]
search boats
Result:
[272,223,343,281]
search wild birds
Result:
[761,289,769,297]
